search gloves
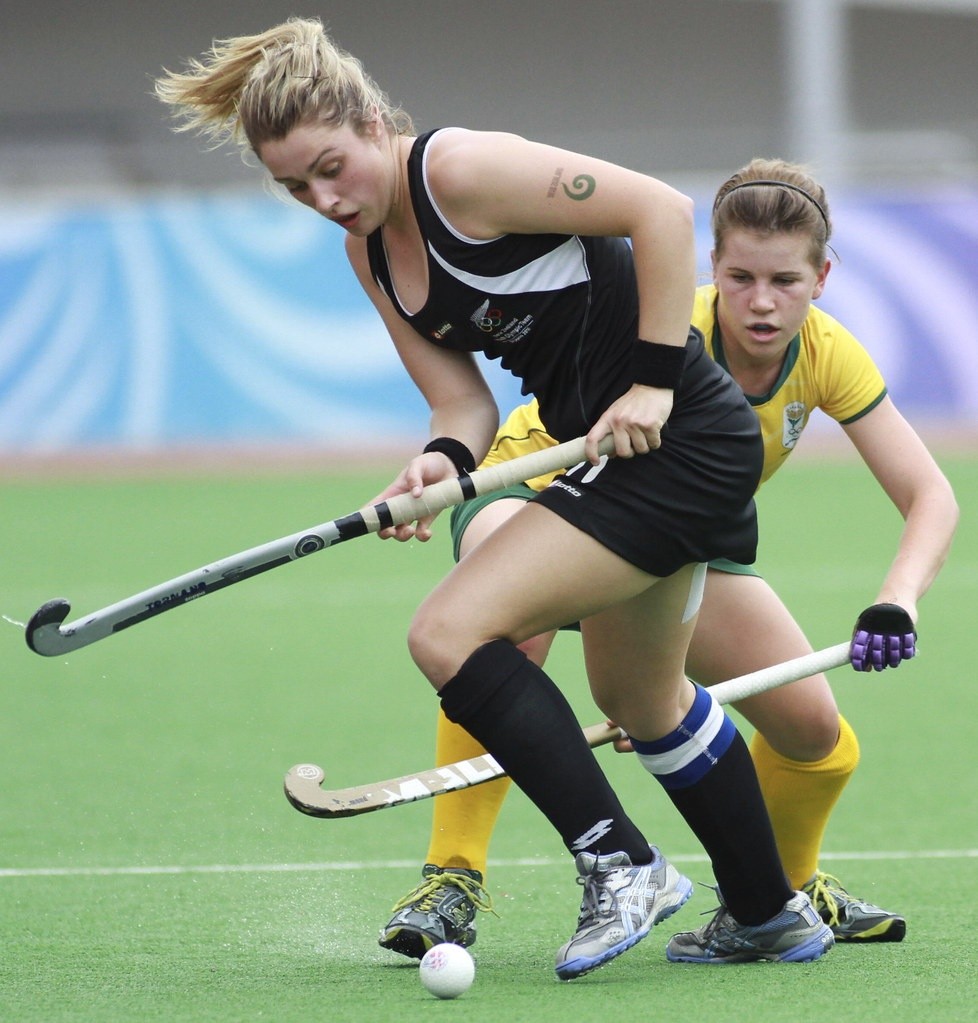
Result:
[848,602,916,674]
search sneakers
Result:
[377,862,503,961]
[554,843,694,980]
[665,881,834,963]
[798,868,907,943]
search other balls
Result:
[418,942,476,998]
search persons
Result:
[378,156,964,960]
[150,16,837,982]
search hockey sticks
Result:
[283,634,852,819]
[25,427,624,659]
